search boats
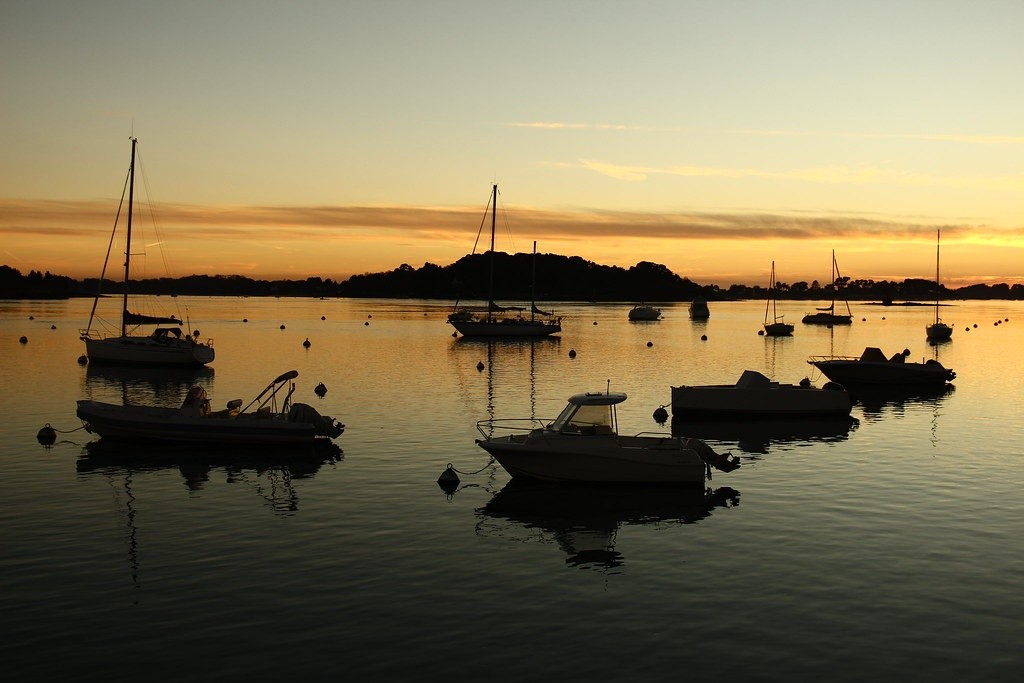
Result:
[688,298,710,319]
[628,303,662,320]
[75,398,345,445]
[669,369,853,418]
[471,391,742,487]
[806,346,956,383]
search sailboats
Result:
[762,260,795,335]
[446,184,562,337]
[76,135,217,364]
[925,229,954,339]
[802,249,854,323]
